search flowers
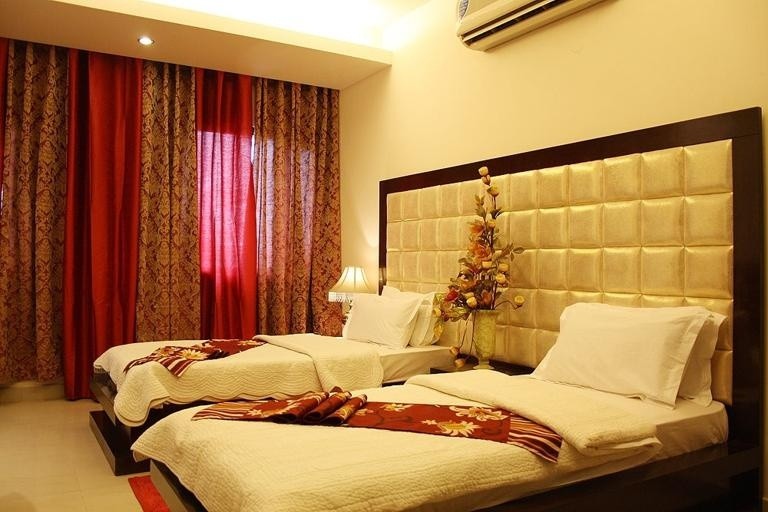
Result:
[432,167,527,369]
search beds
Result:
[88,336,461,476]
[130,351,736,510]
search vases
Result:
[470,308,501,372]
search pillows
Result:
[529,305,724,409]
[342,284,448,349]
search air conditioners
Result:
[455,0,622,56]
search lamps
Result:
[330,265,378,326]
